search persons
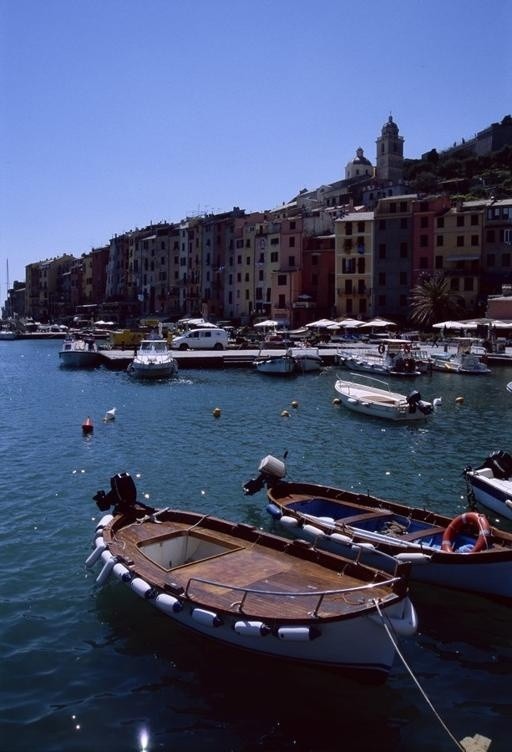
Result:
[431,334,439,348]
[395,332,402,349]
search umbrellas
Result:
[336,318,365,334]
[430,320,477,336]
[252,318,290,332]
[358,317,399,331]
[305,318,339,336]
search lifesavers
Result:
[441,512,490,553]
[378,344,385,354]
[405,347,411,353]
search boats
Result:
[58,337,101,372]
[239,455,512,607]
[459,448,512,527]
[84,471,418,678]
[128,338,180,379]
[0,322,16,341]
[333,374,434,422]
[254,335,495,378]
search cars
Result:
[51,324,60,332]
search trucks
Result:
[170,328,228,350]
